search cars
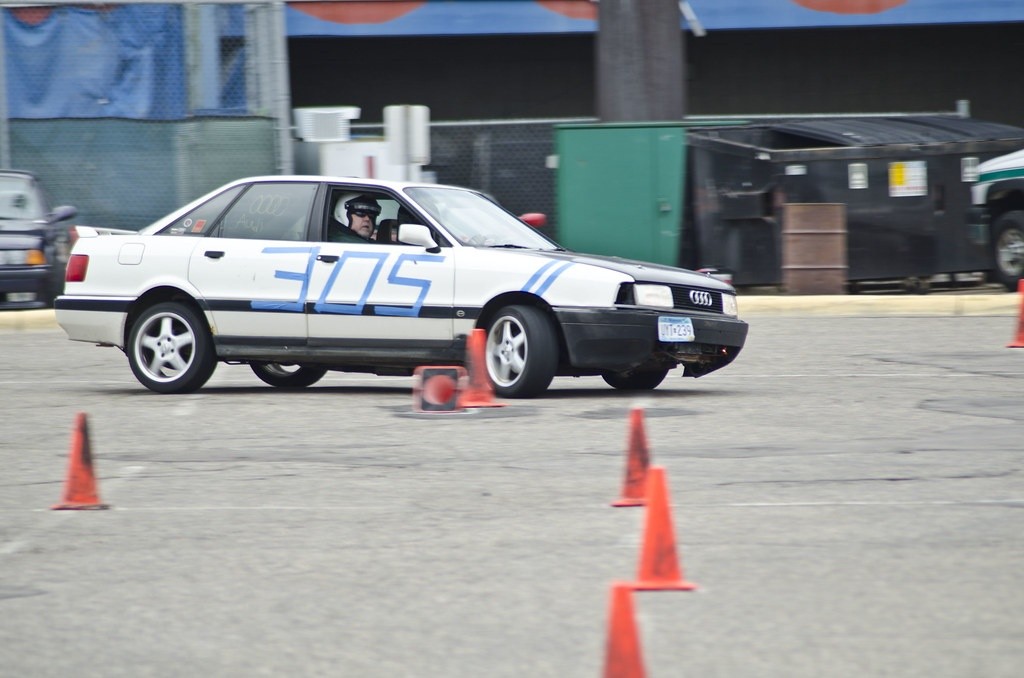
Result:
[964,150,1024,290]
[0,169,77,311]
[54,172,748,401]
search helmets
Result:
[333,193,381,228]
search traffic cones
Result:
[454,329,506,407]
[412,366,467,414]
[49,412,112,511]
[1005,279,1024,347]
[599,584,648,678]
[612,407,651,506]
[627,467,693,592]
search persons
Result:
[333,193,382,244]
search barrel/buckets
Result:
[781,203,848,295]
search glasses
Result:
[350,210,376,219]
